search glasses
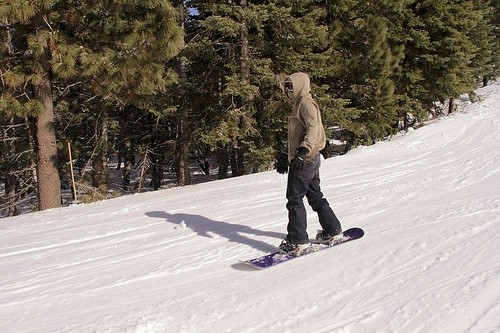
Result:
[283,80,294,93]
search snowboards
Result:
[238,228,365,269]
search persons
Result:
[272,72,343,255]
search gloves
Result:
[290,152,305,172]
[276,153,287,173]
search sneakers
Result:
[280,239,309,253]
[315,231,343,244]
